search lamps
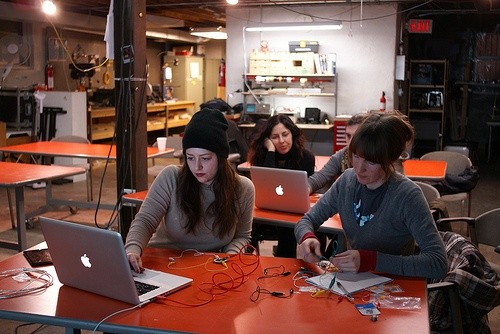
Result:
[189,27,226,39]
[245,21,343,31]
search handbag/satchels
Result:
[200,98,230,112]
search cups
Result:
[157,137,167,152]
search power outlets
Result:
[122,189,136,207]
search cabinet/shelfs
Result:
[35,90,88,183]
[407,58,448,153]
[88,101,195,172]
[241,72,338,123]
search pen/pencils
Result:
[336,280,355,303]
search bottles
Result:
[45,62,54,91]
[80,81,85,92]
[379,91,386,112]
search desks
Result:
[237,124,335,154]
[0,142,175,221]
[237,156,448,180]
[0,241,430,334]
[122,185,344,238]
[0,161,86,253]
[0,120,31,164]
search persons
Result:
[224,114,406,258]
[294,116,448,280]
[125,109,255,272]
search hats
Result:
[182,108,230,159]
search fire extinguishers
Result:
[218,58,225,87]
[380,91,386,112]
[46,63,55,90]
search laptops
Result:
[250,166,316,214]
[36,216,193,305]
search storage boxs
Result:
[0,87,36,132]
[248,51,316,75]
[413,118,441,140]
[288,41,320,53]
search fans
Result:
[0,33,31,90]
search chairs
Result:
[420,146,500,334]
